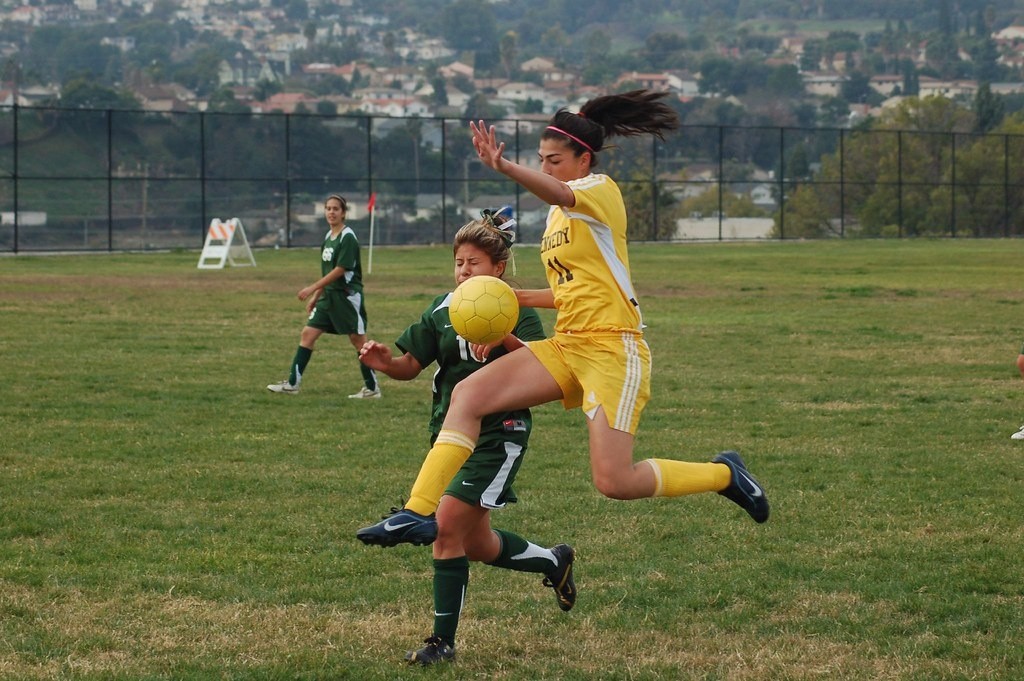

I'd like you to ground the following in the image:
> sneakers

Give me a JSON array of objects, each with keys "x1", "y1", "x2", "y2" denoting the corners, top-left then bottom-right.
[
  {"x1": 348, "y1": 387, "x2": 382, "y2": 399},
  {"x1": 267, "y1": 380, "x2": 299, "y2": 395},
  {"x1": 710, "y1": 449, "x2": 769, "y2": 523},
  {"x1": 403, "y1": 636, "x2": 456, "y2": 665},
  {"x1": 357, "y1": 494, "x2": 438, "y2": 548},
  {"x1": 542, "y1": 543, "x2": 576, "y2": 612}
]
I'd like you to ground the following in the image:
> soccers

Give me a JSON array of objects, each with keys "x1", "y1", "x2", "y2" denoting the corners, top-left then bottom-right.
[{"x1": 448, "y1": 275, "x2": 521, "y2": 346}]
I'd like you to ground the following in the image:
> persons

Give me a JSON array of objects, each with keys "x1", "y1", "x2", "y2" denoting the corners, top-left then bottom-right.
[
  {"x1": 359, "y1": 211, "x2": 577, "y2": 668},
  {"x1": 357, "y1": 88, "x2": 771, "y2": 548},
  {"x1": 266, "y1": 194, "x2": 382, "y2": 398}
]
